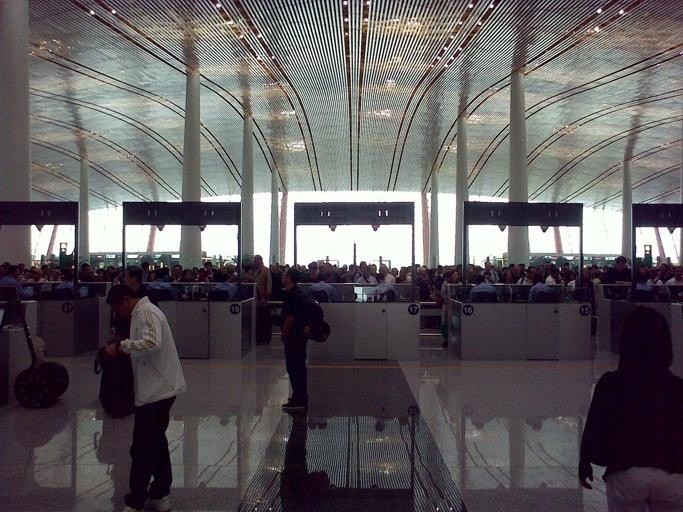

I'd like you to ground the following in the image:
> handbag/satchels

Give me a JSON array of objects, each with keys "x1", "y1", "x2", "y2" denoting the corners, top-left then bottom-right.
[{"x1": 99, "y1": 352, "x2": 134, "y2": 419}]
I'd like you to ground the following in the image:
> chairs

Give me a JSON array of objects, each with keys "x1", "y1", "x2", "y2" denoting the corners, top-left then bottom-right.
[
  {"x1": 535, "y1": 290, "x2": 560, "y2": 304},
  {"x1": 471, "y1": 291, "x2": 496, "y2": 303},
  {"x1": 379, "y1": 289, "x2": 396, "y2": 304},
  {"x1": 0, "y1": 283, "x2": 20, "y2": 303},
  {"x1": 146, "y1": 287, "x2": 177, "y2": 301},
  {"x1": 637, "y1": 289, "x2": 653, "y2": 301},
  {"x1": 207, "y1": 288, "x2": 230, "y2": 301},
  {"x1": 41, "y1": 287, "x2": 72, "y2": 301},
  {"x1": 310, "y1": 289, "x2": 328, "y2": 302}
]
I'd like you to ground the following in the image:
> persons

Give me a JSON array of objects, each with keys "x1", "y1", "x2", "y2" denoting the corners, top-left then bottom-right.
[
  {"x1": 0, "y1": 255, "x2": 124, "y2": 298},
  {"x1": 145, "y1": 254, "x2": 275, "y2": 346},
  {"x1": 105, "y1": 283, "x2": 187, "y2": 512},
  {"x1": 579, "y1": 304, "x2": 683, "y2": 512},
  {"x1": 114, "y1": 266, "x2": 159, "y2": 336},
  {"x1": 272, "y1": 254, "x2": 683, "y2": 303},
  {"x1": 279, "y1": 410, "x2": 307, "y2": 512},
  {"x1": 280, "y1": 272, "x2": 311, "y2": 409}
]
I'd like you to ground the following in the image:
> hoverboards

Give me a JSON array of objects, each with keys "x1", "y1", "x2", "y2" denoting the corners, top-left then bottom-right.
[
  {"x1": 7, "y1": 290, "x2": 69, "y2": 409},
  {"x1": 6, "y1": 401, "x2": 68, "y2": 508}
]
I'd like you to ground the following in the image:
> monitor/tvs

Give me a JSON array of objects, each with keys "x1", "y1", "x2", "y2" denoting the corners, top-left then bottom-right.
[
  {"x1": 77, "y1": 284, "x2": 91, "y2": 298},
  {"x1": 22, "y1": 284, "x2": 36, "y2": 299}
]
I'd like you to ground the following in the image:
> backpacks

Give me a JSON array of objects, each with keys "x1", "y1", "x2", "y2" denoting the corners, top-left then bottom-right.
[{"x1": 302, "y1": 298, "x2": 330, "y2": 343}]
